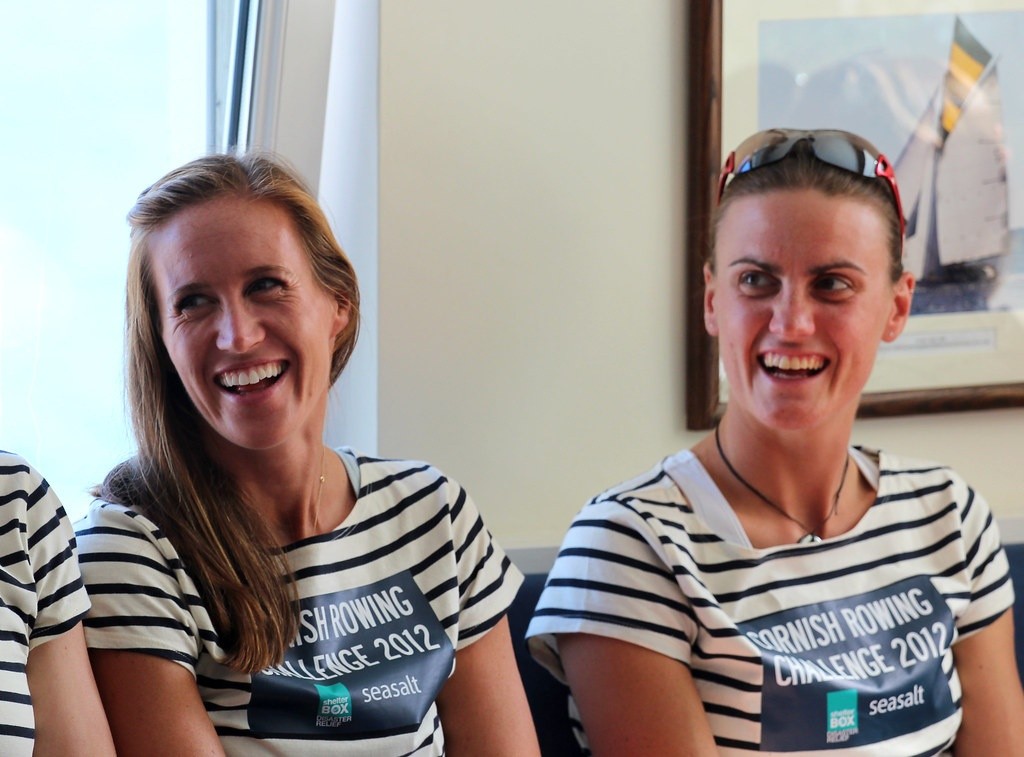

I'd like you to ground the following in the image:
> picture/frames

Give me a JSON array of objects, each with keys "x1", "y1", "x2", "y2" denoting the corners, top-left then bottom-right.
[{"x1": 685, "y1": 0, "x2": 1024, "y2": 431}]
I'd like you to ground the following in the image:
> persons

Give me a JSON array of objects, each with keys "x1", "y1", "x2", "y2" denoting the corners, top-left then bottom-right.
[
  {"x1": 71, "y1": 150, "x2": 541, "y2": 757},
  {"x1": 0, "y1": 449, "x2": 117, "y2": 757},
  {"x1": 521, "y1": 129, "x2": 1024, "y2": 757}
]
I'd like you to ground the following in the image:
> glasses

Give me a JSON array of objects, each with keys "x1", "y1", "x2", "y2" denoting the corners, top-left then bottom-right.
[{"x1": 716, "y1": 128, "x2": 905, "y2": 244}]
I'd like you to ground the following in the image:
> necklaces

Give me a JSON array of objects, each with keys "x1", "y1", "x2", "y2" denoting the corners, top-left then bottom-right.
[
  {"x1": 716, "y1": 419, "x2": 849, "y2": 547},
  {"x1": 253, "y1": 449, "x2": 327, "y2": 544}
]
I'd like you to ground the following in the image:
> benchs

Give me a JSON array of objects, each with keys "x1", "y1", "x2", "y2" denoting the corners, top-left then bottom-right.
[{"x1": 508, "y1": 544, "x2": 1024, "y2": 757}]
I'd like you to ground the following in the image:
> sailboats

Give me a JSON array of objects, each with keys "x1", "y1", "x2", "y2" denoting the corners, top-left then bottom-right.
[{"x1": 870, "y1": 11, "x2": 1011, "y2": 292}]
